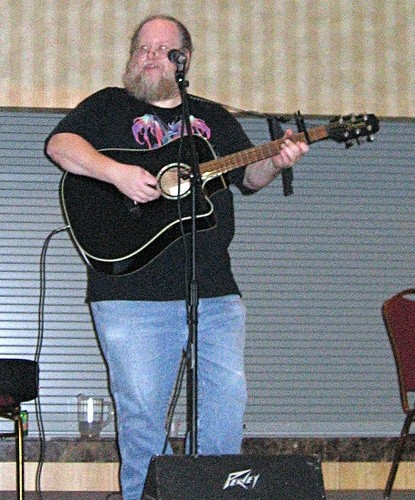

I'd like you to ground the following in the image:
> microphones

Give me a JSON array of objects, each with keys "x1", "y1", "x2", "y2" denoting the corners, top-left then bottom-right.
[{"x1": 167, "y1": 49, "x2": 187, "y2": 64}]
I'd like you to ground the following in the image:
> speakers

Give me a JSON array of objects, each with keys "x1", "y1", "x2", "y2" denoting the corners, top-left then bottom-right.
[{"x1": 139, "y1": 453, "x2": 326, "y2": 500}]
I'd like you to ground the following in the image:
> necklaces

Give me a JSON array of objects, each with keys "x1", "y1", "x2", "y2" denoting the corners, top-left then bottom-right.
[{"x1": 149, "y1": 104, "x2": 181, "y2": 144}]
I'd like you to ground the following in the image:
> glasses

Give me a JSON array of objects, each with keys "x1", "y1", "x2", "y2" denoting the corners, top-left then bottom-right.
[{"x1": 131, "y1": 47, "x2": 171, "y2": 59}]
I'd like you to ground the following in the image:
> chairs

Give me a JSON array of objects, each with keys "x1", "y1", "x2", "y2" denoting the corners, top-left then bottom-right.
[
  {"x1": 0, "y1": 358, "x2": 39, "y2": 500},
  {"x1": 381, "y1": 289, "x2": 415, "y2": 500}
]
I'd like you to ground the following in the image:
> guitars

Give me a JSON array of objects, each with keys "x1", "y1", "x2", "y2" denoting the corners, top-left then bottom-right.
[{"x1": 60, "y1": 112, "x2": 379, "y2": 278}]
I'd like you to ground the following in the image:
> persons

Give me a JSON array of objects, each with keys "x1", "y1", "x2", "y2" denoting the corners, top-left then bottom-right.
[{"x1": 43, "y1": 11, "x2": 311, "y2": 500}]
